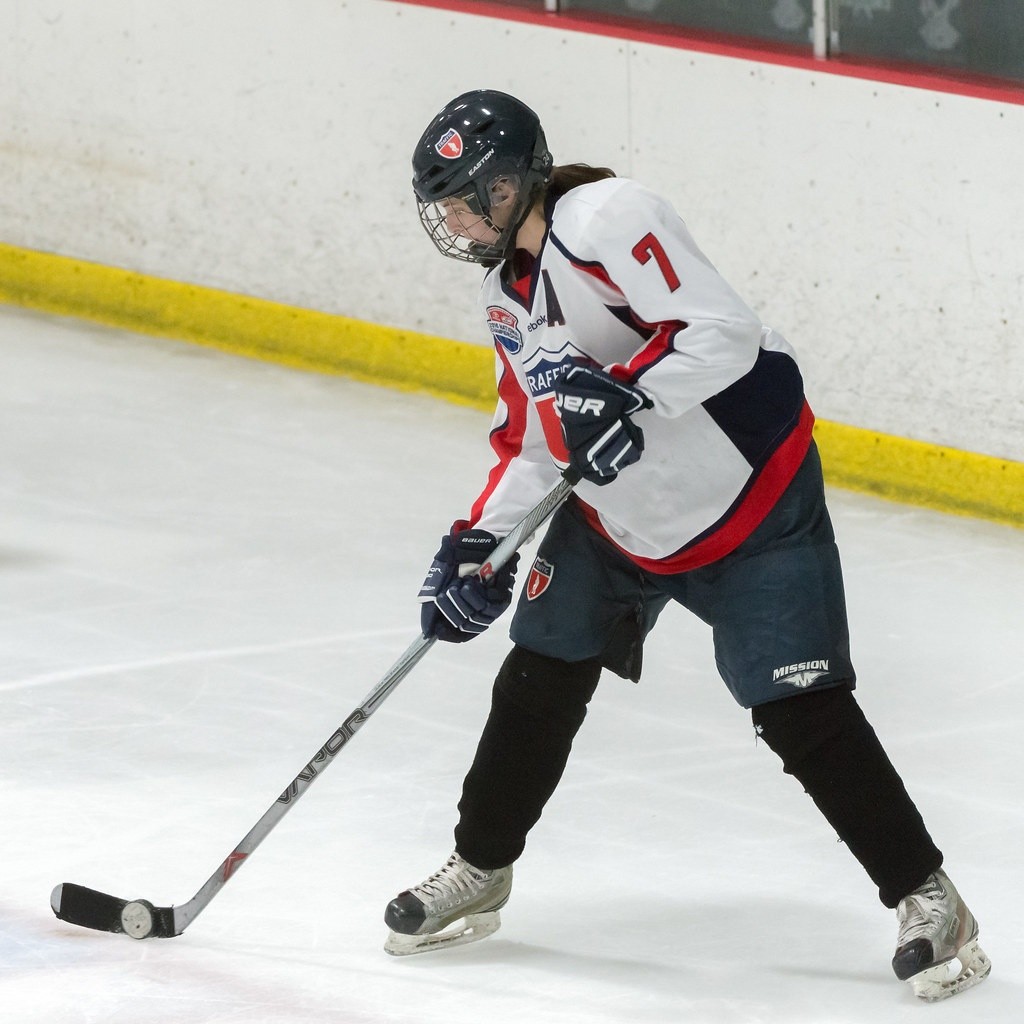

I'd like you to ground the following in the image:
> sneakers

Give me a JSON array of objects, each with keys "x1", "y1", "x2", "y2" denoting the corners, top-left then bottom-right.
[
  {"x1": 384, "y1": 854, "x2": 515, "y2": 955},
  {"x1": 892, "y1": 869, "x2": 993, "y2": 1001}
]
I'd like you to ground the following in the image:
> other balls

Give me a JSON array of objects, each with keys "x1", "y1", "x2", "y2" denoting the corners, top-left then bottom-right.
[{"x1": 119, "y1": 899, "x2": 160, "y2": 940}]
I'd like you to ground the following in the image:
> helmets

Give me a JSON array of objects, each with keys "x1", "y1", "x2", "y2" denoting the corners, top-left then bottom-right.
[{"x1": 412, "y1": 91, "x2": 551, "y2": 266}]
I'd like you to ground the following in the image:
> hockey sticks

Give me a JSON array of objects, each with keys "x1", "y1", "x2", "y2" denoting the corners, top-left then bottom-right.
[{"x1": 48, "y1": 458, "x2": 598, "y2": 939}]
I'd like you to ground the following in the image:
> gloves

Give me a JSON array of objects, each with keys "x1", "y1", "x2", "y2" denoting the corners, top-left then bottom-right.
[
  {"x1": 419, "y1": 530, "x2": 519, "y2": 645},
  {"x1": 555, "y1": 356, "x2": 654, "y2": 484}
]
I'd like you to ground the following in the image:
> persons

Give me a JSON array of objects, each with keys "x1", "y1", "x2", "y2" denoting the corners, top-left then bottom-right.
[{"x1": 381, "y1": 90, "x2": 993, "y2": 1002}]
